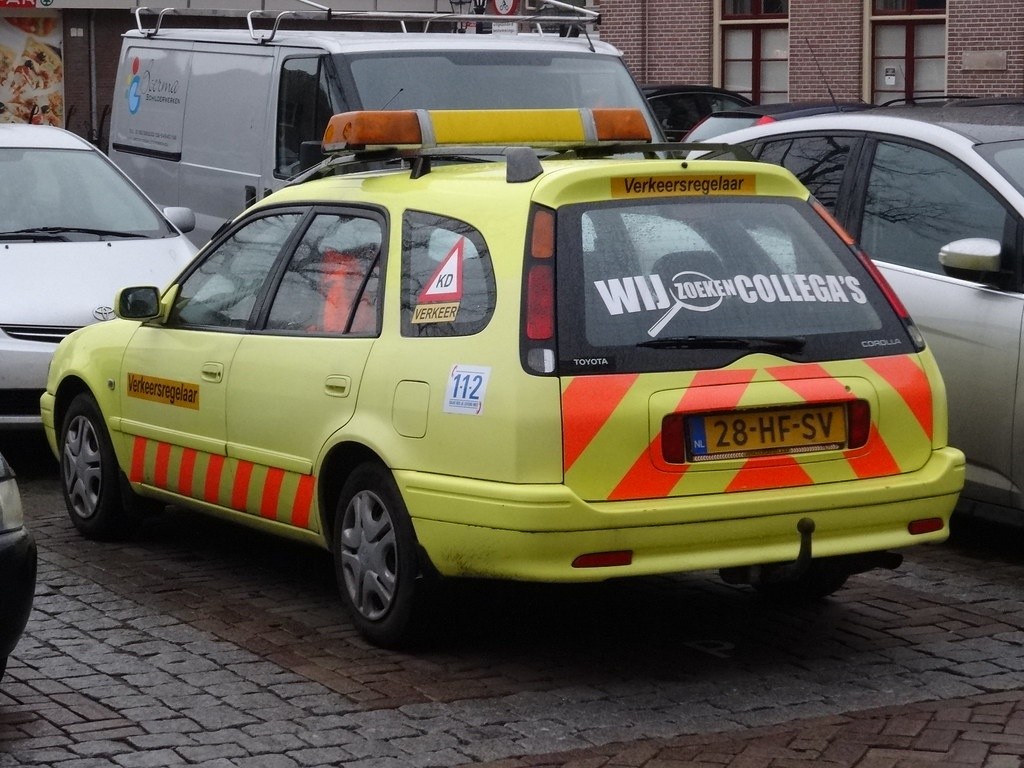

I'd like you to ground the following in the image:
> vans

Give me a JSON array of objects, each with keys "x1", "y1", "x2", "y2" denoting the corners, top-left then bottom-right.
[{"x1": 99, "y1": 0, "x2": 670, "y2": 251}]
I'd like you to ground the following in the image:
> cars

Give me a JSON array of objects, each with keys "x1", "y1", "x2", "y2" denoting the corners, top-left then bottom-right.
[
  {"x1": 680, "y1": 93, "x2": 1024, "y2": 534},
  {"x1": 39, "y1": 103, "x2": 969, "y2": 650},
  {"x1": 0, "y1": 119, "x2": 210, "y2": 434},
  {"x1": 634, "y1": 83, "x2": 759, "y2": 151}
]
